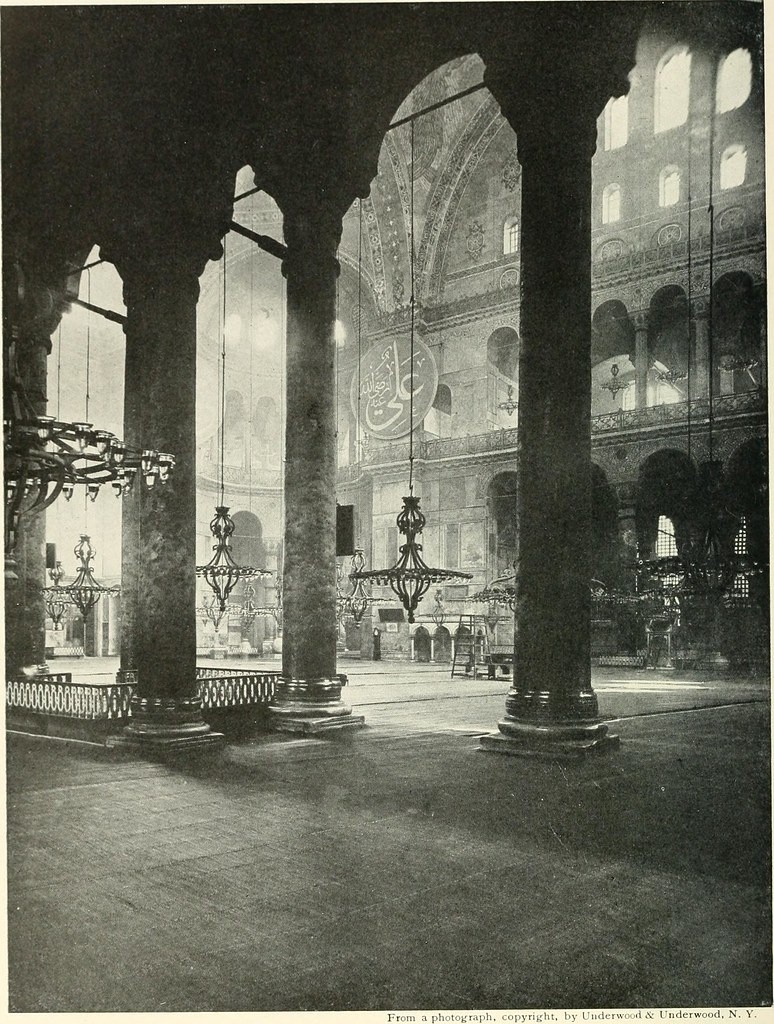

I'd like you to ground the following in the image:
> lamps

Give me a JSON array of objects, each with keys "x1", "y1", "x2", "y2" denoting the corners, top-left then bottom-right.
[
  {"x1": 587, "y1": 36, "x2": 770, "y2": 651},
  {"x1": 45, "y1": 267, "x2": 120, "y2": 631},
  {"x1": 336, "y1": 119, "x2": 515, "y2": 633},
  {"x1": 1, "y1": 322, "x2": 178, "y2": 581},
  {"x1": 195, "y1": 235, "x2": 285, "y2": 634}
]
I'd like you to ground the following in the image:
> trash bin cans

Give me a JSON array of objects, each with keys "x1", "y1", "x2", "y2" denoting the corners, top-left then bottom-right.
[{"x1": 488, "y1": 664, "x2": 503, "y2": 680}]
